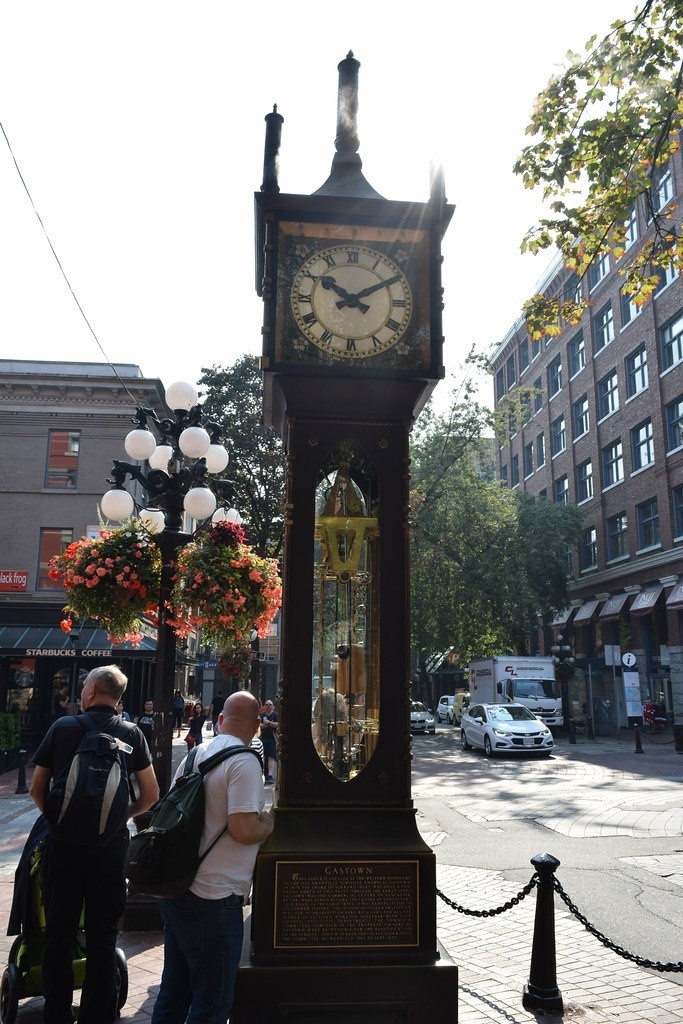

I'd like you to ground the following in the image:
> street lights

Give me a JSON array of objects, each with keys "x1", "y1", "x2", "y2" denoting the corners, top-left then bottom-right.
[
  {"x1": 548, "y1": 636, "x2": 580, "y2": 744},
  {"x1": 98, "y1": 381, "x2": 244, "y2": 930}
]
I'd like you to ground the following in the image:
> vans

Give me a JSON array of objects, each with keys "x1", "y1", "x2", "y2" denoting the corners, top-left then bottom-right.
[{"x1": 453, "y1": 693, "x2": 469, "y2": 724}]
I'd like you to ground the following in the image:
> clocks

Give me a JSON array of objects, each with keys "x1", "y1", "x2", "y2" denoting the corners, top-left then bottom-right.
[{"x1": 289, "y1": 243, "x2": 414, "y2": 359}]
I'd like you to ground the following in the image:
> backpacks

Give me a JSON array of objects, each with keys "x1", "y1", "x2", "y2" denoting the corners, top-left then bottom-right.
[
  {"x1": 52, "y1": 712, "x2": 138, "y2": 851},
  {"x1": 124, "y1": 745, "x2": 264, "y2": 897}
]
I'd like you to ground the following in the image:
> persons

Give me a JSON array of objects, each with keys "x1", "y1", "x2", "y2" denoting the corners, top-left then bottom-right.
[
  {"x1": 648, "y1": 699, "x2": 669, "y2": 721},
  {"x1": 29, "y1": 664, "x2": 162, "y2": 1024},
  {"x1": 123, "y1": 688, "x2": 280, "y2": 1024}
]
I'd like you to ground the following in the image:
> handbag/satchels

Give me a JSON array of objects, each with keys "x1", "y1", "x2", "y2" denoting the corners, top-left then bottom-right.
[{"x1": 184, "y1": 734, "x2": 197, "y2": 744}]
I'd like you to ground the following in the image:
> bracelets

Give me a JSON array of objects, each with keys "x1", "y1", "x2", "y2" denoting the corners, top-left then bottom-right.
[{"x1": 266, "y1": 720, "x2": 271, "y2": 725}]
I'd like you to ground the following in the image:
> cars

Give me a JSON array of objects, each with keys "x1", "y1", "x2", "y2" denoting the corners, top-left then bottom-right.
[
  {"x1": 571, "y1": 701, "x2": 587, "y2": 734},
  {"x1": 459, "y1": 703, "x2": 554, "y2": 759},
  {"x1": 435, "y1": 695, "x2": 455, "y2": 724},
  {"x1": 410, "y1": 701, "x2": 435, "y2": 735}
]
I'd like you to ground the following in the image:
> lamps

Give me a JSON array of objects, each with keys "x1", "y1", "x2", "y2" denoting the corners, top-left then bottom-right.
[{"x1": 70, "y1": 631, "x2": 81, "y2": 641}]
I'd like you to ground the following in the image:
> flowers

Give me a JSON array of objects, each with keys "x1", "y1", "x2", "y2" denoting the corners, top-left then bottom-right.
[{"x1": 47, "y1": 503, "x2": 283, "y2": 683}]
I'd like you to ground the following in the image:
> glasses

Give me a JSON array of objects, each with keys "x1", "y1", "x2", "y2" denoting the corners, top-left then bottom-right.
[{"x1": 265, "y1": 704, "x2": 273, "y2": 706}]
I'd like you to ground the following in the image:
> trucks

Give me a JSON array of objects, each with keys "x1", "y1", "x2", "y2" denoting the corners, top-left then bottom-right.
[{"x1": 468, "y1": 658, "x2": 565, "y2": 740}]
